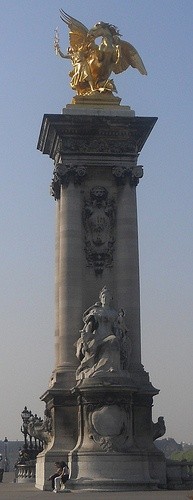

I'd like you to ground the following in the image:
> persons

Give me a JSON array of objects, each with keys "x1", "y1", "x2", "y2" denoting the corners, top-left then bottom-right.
[
  {"x1": 54, "y1": 44, "x2": 91, "y2": 83},
  {"x1": 76, "y1": 285, "x2": 130, "y2": 373},
  {"x1": 51, "y1": 461, "x2": 69, "y2": 493},
  {"x1": 47, "y1": 461, "x2": 63, "y2": 492},
  {"x1": 0, "y1": 453, "x2": 8, "y2": 483}
]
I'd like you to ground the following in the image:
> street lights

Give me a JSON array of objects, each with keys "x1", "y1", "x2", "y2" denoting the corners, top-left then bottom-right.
[
  {"x1": 2, "y1": 437, "x2": 9, "y2": 472},
  {"x1": 19, "y1": 406, "x2": 43, "y2": 451}
]
